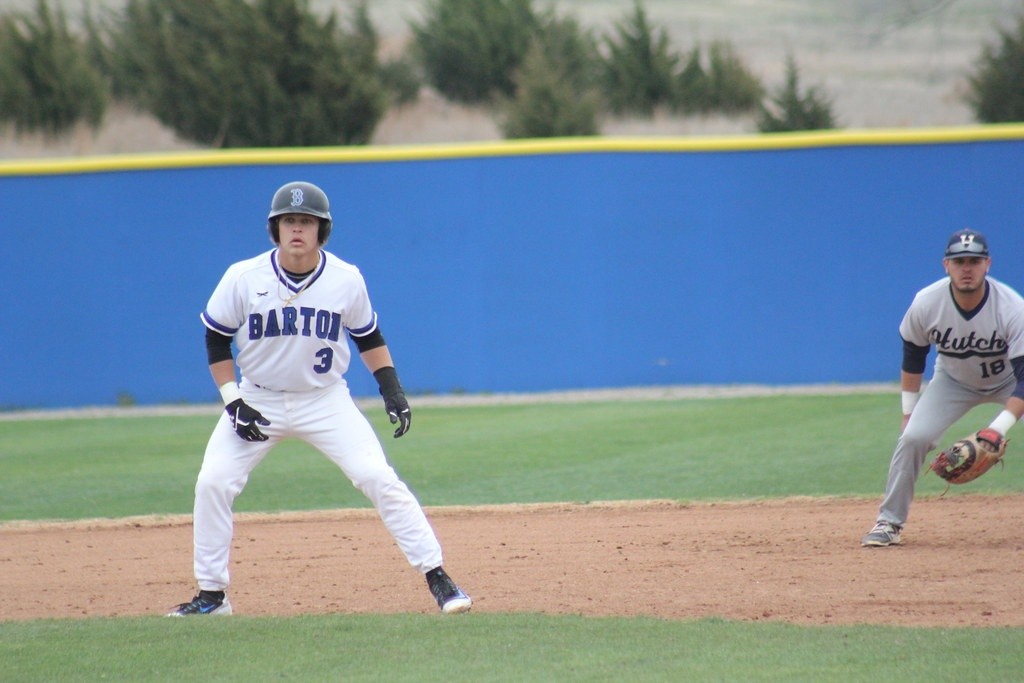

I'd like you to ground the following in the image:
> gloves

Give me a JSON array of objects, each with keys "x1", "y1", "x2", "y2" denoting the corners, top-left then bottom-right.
[
  {"x1": 225, "y1": 398, "x2": 271, "y2": 441},
  {"x1": 385, "y1": 392, "x2": 411, "y2": 439}
]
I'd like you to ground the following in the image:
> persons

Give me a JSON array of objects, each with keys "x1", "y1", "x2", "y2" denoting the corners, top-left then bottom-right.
[
  {"x1": 164, "y1": 181, "x2": 473, "y2": 617},
  {"x1": 860, "y1": 230, "x2": 1024, "y2": 547}
]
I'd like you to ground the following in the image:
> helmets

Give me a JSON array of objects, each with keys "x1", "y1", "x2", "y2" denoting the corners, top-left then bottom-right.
[{"x1": 267, "y1": 181, "x2": 332, "y2": 243}]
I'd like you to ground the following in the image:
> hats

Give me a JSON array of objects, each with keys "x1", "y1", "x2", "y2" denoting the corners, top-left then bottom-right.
[{"x1": 944, "y1": 229, "x2": 989, "y2": 260}]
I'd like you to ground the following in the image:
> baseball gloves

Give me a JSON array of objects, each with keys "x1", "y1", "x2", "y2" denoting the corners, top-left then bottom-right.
[{"x1": 929, "y1": 429, "x2": 1008, "y2": 485}]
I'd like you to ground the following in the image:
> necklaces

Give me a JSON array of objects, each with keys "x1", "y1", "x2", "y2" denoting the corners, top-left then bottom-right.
[{"x1": 277, "y1": 254, "x2": 320, "y2": 308}]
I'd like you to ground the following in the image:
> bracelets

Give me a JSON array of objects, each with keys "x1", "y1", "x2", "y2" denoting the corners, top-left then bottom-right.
[
  {"x1": 219, "y1": 381, "x2": 240, "y2": 406},
  {"x1": 901, "y1": 391, "x2": 919, "y2": 414},
  {"x1": 989, "y1": 410, "x2": 1016, "y2": 436}
]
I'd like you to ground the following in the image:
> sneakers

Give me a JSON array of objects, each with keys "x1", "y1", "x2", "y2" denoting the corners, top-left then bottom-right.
[
  {"x1": 426, "y1": 565, "x2": 471, "y2": 614},
  {"x1": 165, "y1": 591, "x2": 233, "y2": 617},
  {"x1": 860, "y1": 520, "x2": 904, "y2": 548}
]
